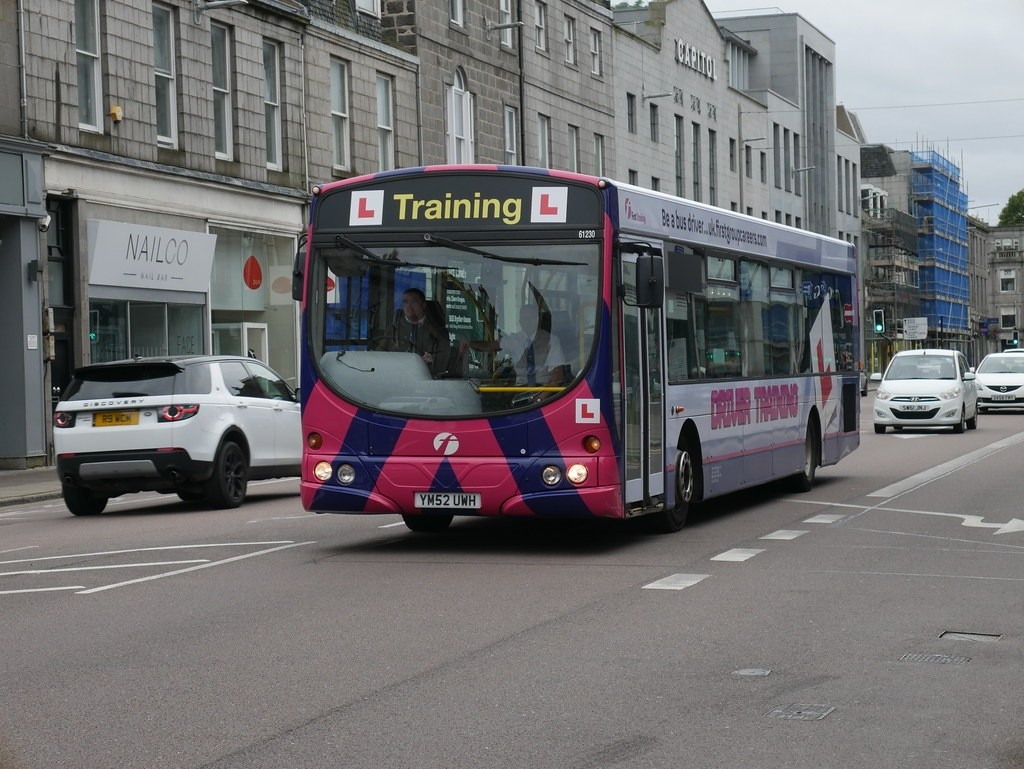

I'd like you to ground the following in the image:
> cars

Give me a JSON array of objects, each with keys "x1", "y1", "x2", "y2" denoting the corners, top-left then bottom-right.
[
  {"x1": 970, "y1": 352, "x2": 1024, "y2": 414},
  {"x1": 870, "y1": 349, "x2": 979, "y2": 434}
]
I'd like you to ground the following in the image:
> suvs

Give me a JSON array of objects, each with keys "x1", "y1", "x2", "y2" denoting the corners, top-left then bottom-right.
[{"x1": 53, "y1": 353, "x2": 304, "y2": 517}]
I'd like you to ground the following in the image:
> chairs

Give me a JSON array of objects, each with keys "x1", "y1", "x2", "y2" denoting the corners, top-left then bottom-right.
[{"x1": 541, "y1": 311, "x2": 577, "y2": 364}]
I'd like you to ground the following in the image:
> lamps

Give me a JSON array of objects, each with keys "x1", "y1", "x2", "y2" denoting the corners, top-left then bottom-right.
[
  {"x1": 741, "y1": 136, "x2": 767, "y2": 143},
  {"x1": 790, "y1": 164, "x2": 815, "y2": 178},
  {"x1": 872, "y1": 309, "x2": 886, "y2": 334},
  {"x1": 640, "y1": 83, "x2": 673, "y2": 107},
  {"x1": 1013, "y1": 331, "x2": 1020, "y2": 345},
  {"x1": 484, "y1": 14, "x2": 525, "y2": 41}
]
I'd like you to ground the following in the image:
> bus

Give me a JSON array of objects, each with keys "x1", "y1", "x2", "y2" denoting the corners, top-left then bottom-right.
[{"x1": 290, "y1": 162, "x2": 862, "y2": 547}]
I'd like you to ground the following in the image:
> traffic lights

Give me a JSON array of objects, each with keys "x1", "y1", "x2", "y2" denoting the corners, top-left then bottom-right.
[
  {"x1": 1012, "y1": 330, "x2": 1019, "y2": 344},
  {"x1": 873, "y1": 310, "x2": 885, "y2": 334}
]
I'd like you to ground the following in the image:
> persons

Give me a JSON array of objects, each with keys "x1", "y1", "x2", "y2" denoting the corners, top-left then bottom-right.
[
  {"x1": 374, "y1": 286, "x2": 452, "y2": 375},
  {"x1": 464, "y1": 304, "x2": 568, "y2": 408}
]
